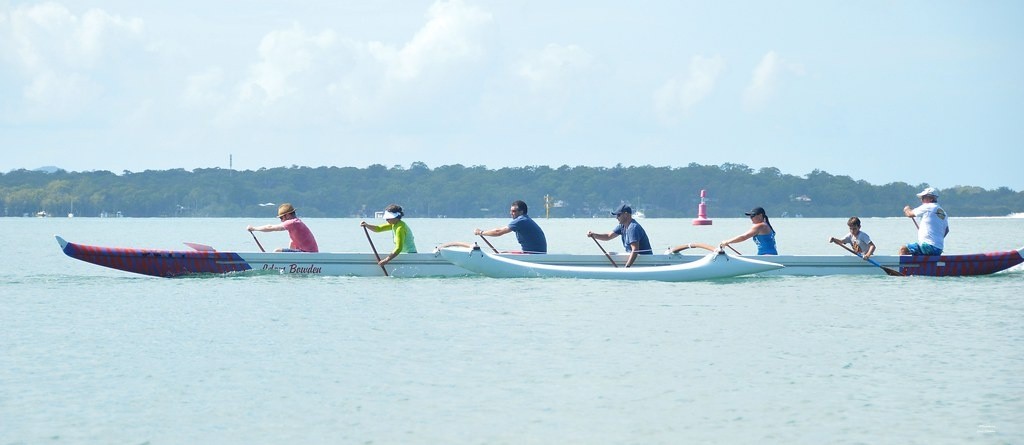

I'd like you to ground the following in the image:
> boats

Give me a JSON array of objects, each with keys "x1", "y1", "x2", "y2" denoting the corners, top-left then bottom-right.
[
  {"x1": 54, "y1": 233, "x2": 1024, "y2": 286},
  {"x1": 432, "y1": 241, "x2": 787, "y2": 281}
]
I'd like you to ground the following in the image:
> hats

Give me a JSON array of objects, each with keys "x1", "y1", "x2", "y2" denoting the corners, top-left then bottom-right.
[
  {"x1": 611, "y1": 204, "x2": 632, "y2": 215},
  {"x1": 745, "y1": 207, "x2": 765, "y2": 216},
  {"x1": 383, "y1": 210, "x2": 401, "y2": 219},
  {"x1": 277, "y1": 203, "x2": 296, "y2": 217},
  {"x1": 916, "y1": 187, "x2": 939, "y2": 199}
]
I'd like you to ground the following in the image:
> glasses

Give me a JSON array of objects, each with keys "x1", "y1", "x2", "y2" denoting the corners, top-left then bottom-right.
[
  {"x1": 280, "y1": 217, "x2": 284, "y2": 219},
  {"x1": 511, "y1": 210, "x2": 520, "y2": 213},
  {"x1": 617, "y1": 214, "x2": 623, "y2": 217},
  {"x1": 850, "y1": 226, "x2": 860, "y2": 231},
  {"x1": 751, "y1": 215, "x2": 757, "y2": 219},
  {"x1": 921, "y1": 196, "x2": 930, "y2": 199}
]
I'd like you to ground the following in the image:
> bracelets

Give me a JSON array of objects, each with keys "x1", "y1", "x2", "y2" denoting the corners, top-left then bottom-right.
[
  {"x1": 626, "y1": 266, "x2": 630, "y2": 267},
  {"x1": 481, "y1": 231, "x2": 483, "y2": 234},
  {"x1": 866, "y1": 255, "x2": 868, "y2": 258}
]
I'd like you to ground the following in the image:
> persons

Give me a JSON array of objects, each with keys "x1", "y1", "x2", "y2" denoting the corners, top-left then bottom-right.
[
  {"x1": 473, "y1": 200, "x2": 547, "y2": 254},
  {"x1": 586, "y1": 204, "x2": 653, "y2": 268},
  {"x1": 899, "y1": 187, "x2": 949, "y2": 256},
  {"x1": 360, "y1": 204, "x2": 417, "y2": 268},
  {"x1": 720, "y1": 206, "x2": 778, "y2": 255},
  {"x1": 830, "y1": 218, "x2": 876, "y2": 260},
  {"x1": 247, "y1": 203, "x2": 318, "y2": 252}
]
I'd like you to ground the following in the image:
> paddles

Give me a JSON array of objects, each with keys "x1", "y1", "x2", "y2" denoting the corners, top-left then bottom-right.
[
  {"x1": 590, "y1": 235, "x2": 618, "y2": 267},
  {"x1": 912, "y1": 216, "x2": 919, "y2": 229},
  {"x1": 481, "y1": 235, "x2": 498, "y2": 253},
  {"x1": 727, "y1": 244, "x2": 742, "y2": 256},
  {"x1": 249, "y1": 229, "x2": 266, "y2": 252},
  {"x1": 833, "y1": 239, "x2": 904, "y2": 276},
  {"x1": 363, "y1": 225, "x2": 388, "y2": 276}
]
